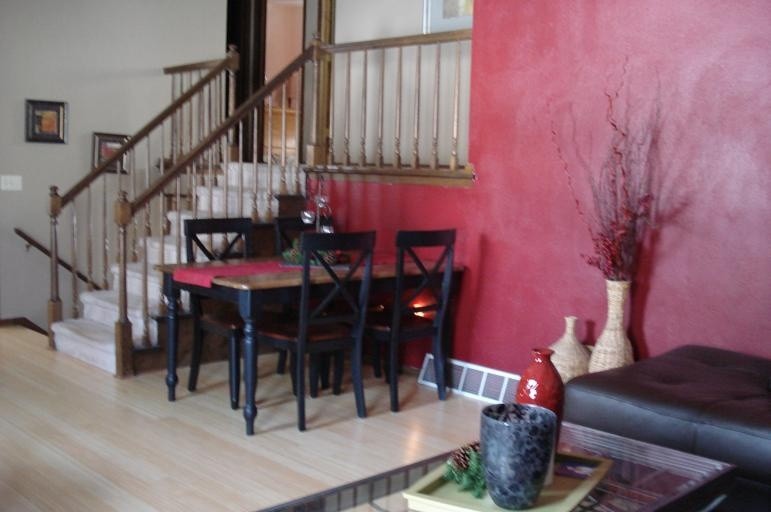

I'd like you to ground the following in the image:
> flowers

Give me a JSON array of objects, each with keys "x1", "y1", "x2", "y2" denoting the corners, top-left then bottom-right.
[{"x1": 552, "y1": 56, "x2": 670, "y2": 279}]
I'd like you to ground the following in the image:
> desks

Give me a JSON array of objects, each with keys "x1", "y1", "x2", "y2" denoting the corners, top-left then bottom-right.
[
  {"x1": 255, "y1": 418, "x2": 740, "y2": 512},
  {"x1": 153, "y1": 252, "x2": 470, "y2": 412}
]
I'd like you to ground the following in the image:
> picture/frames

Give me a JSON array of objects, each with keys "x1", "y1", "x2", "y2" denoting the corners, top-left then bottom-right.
[
  {"x1": 25, "y1": 98, "x2": 71, "y2": 144},
  {"x1": 92, "y1": 132, "x2": 133, "y2": 175},
  {"x1": 422, "y1": 0, "x2": 475, "y2": 37}
]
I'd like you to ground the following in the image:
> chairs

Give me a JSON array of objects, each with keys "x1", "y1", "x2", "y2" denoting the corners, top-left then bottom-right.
[
  {"x1": 180, "y1": 214, "x2": 301, "y2": 410},
  {"x1": 252, "y1": 228, "x2": 379, "y2": 429},
  {"x1": 272, "y1": 213, "x2": 376, "y2": 376},
  {"x1": 332, "y1": 222, "x2": 461, "y2": 410}
]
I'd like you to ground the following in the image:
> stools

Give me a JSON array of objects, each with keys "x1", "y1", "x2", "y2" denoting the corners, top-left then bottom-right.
[{"x1": 563, "y1": 343, "x2": 771, "y2": 510}]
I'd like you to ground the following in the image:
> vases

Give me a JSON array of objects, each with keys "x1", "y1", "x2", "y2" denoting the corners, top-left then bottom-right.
[
  {"x1": 548, "y1": 312, "x2": 593, "y2": 385},
  {"x1": 588, "y1": 277, "x2": 637, "y2": 374},
  {"x1": 517, "y1": 346, "x2": 567, "y2": 420}
]
